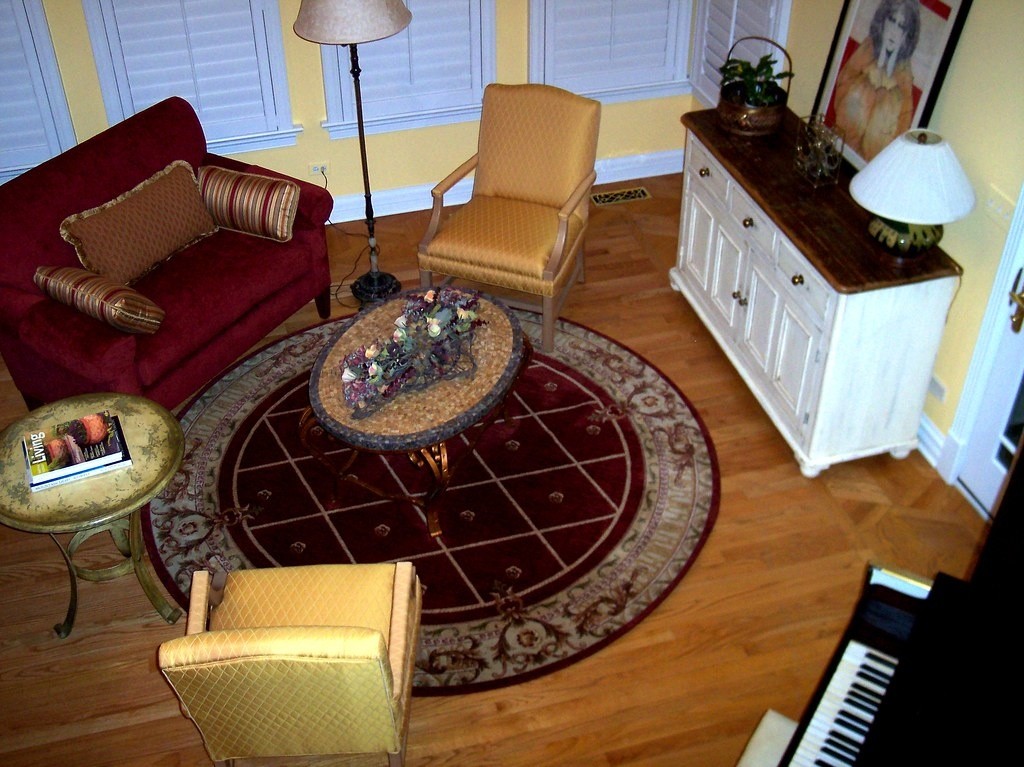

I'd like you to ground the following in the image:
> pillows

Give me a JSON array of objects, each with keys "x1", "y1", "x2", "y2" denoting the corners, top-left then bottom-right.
[
  {"x1": 33, "y1": 265, "x2": 167, "y2": 336},
  {"x1": 197, "y1": 164, "x2": 300, "y2": 243},
  {"x1": 59, "y1": 160, "x2": 219, "y2": 285}
]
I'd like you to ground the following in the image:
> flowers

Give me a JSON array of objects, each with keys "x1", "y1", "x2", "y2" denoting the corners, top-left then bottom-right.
[{"x1": 338, "y1": 284, "x2": 490, "y2": 420}]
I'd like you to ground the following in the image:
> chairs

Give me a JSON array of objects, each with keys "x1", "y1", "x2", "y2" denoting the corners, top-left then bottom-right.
[
  {"x1": 417, "y1": 82, "x2": 602, "y2": 352},
  {"x1": 157, "y1": 561, "x2": 423, "y2": 767}
]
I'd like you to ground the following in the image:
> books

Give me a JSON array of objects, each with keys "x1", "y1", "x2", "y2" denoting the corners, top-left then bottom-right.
[{"x1": 22, "y1": 409, "x2": 133, "y2": 493}]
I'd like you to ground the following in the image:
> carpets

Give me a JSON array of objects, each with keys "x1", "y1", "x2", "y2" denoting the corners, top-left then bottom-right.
[{"x1": 126, "y1": 299, "x2": 721, "y2": 697}]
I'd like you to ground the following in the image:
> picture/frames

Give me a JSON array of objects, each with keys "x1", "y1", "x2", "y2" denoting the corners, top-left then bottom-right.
[{"x1": 808, "y1": 0, "x2": 974, "y2": 180}]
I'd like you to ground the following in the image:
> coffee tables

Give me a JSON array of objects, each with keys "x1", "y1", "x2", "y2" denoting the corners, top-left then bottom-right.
[{"x1": 310, "y1": 286, "x2": 524, "y2": 538}]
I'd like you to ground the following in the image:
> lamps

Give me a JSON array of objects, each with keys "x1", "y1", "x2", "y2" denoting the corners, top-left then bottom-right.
[
  {"x1": 292, "y1": 0, "x2": 412, "y2": 305},
  {"x1": 849, "y1": 128, "x2": 977, "y2": 256}
]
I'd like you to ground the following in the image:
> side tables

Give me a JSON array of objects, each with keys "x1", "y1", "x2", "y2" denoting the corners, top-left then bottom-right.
[{"x1": 0, "y1": 393, "x2": 183, "y2": 639}]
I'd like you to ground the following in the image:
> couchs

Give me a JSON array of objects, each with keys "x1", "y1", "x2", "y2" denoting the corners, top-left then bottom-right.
[{"x1": 0, "y1": 94, "x2": 332, "y2": 412}]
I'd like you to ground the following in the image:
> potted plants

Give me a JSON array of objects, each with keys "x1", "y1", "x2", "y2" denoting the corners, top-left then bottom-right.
[{"x1": 716, "y1": 35, "x2": 795, "y2": 138}]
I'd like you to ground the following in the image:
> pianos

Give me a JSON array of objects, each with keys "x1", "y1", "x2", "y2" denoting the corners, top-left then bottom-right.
[{"x1": 775, "y1": 421, "x2": 1024, "y2": 767}]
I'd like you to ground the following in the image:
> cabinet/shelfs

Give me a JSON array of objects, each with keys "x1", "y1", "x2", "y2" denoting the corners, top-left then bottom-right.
[{"x1": 669, "y1": 105, "x2": 963, "y2": 477}]
